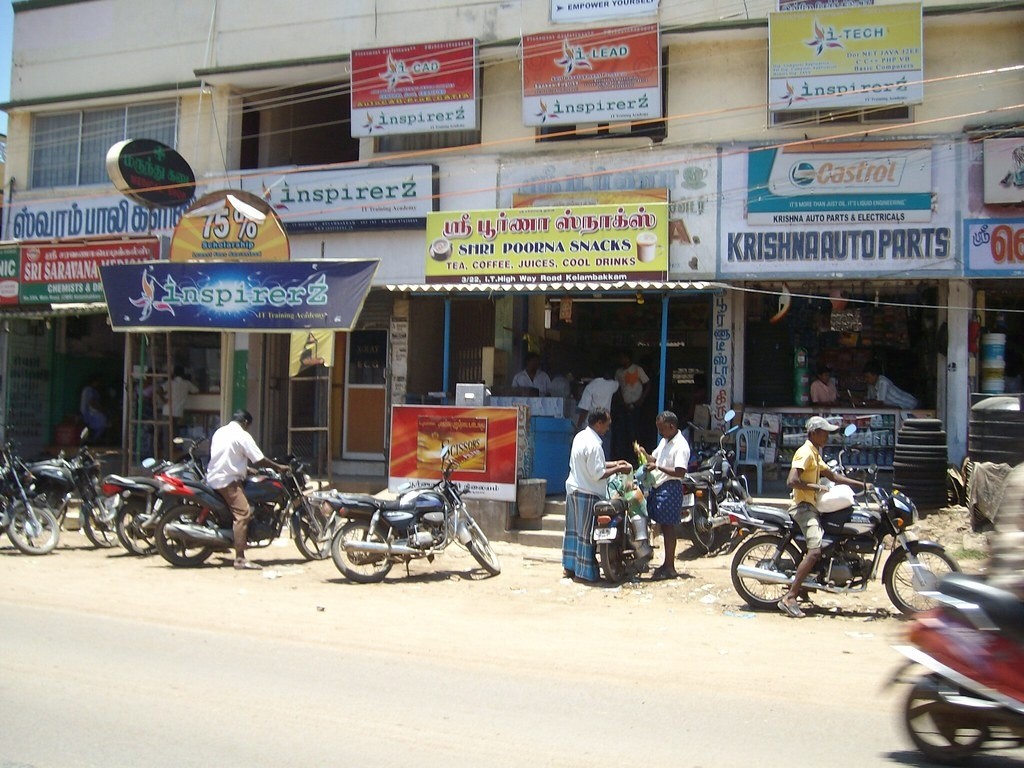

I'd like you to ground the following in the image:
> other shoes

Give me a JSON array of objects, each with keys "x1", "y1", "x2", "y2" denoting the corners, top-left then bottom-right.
[{"x1": 563, "y1": 570, "x2": 592, "y2": 583}]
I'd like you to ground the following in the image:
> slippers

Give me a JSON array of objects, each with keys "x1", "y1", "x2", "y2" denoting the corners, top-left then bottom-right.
[
  {"x1": 778, "y1": 595, "x2": 805, "y2": 617},
  {"x1": 234, "y1": 559, "x2": 262, "y2": 569},
  {"x1": 650, "y1": 564, "x2": 679, "y2": 581}
]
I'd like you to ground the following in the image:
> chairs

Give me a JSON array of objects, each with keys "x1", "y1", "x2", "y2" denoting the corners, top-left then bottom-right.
[{"x1": 732, "y1": 426, "x2": 769, "y2": 497}]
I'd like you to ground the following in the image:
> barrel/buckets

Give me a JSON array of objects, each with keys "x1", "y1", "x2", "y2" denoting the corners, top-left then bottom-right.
[
  {"x1": 793, "y1": 345, "x2": 809, "y2": 408},
  {"x1": 982, "y1": 333, "x2": 1006, "y2": 394}
]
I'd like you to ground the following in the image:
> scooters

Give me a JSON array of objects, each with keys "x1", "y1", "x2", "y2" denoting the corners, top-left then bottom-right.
[{"x1": 881, "y1": 567, "x2": 1024, "y2": 762}]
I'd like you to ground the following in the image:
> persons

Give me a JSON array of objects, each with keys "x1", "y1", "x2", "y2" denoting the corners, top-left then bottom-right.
[
  {"x1": 992, "y1": 461, "x2": 1024, "y2": 600},
  {"x1": 136, "y1": 367, "x2": 199, "y2": 458},
  {"x1": 778, "y1": 415, "x2": 874, "y2": 617},
  {"x1": 82, "y1": 373, "x2": 111, "y2": 442},
  {"x1": 513, "y1": 350, "x2": 707, "y2": 460},
  {"x1": 635, "y1": 410, "x2": 690, "y2": 579},
  {"x1": 863, "y1": 360, "x2": 920, "y2": 411},
  {"x1": 811, "y1": 366, "x2": 841, "y2": 407},
  {"x1": 560, "y1": 407, "x2": 632, "y2": 583},
  {"x1": 206, "y1": 408, "x2": 292, "y2": 570}
]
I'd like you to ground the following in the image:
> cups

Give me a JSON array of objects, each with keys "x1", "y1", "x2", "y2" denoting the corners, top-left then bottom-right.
[
  {"x1": 637, "y1": 232, "x2": 657, "y2": 262},
  {"x1": 432, "y1": 239, "x2": 450, "y2": 255}
]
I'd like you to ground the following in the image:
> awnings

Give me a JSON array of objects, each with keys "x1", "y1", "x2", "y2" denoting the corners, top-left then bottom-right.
[{"x1": 384, "y1": 282, "x2": 724, "y2": 293}]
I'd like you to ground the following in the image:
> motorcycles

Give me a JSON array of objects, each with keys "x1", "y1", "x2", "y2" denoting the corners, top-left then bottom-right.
[
  {"x1": 312, "y1": 446, "x2": 500, "y2": 586},
  {"x1": 679, "y1": 410, "x2": 757, "y2": 552},
  {"x1": 2, "y1": 426, "x2": 335, "y2": 568},
  {"x1": 718, "y1": 461, "x2": 959, "y2": 616},
  {"x1": 821, "y1": 423, "x2": 866, "y2": 491},
  {"x1": 592, "y1": 471, "x2": 660, "y2": 583}
]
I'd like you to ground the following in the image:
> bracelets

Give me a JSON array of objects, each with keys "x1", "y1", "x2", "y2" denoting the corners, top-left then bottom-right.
[{"x1": 615, "y1": 461, "x2": 617, "y2": 467}]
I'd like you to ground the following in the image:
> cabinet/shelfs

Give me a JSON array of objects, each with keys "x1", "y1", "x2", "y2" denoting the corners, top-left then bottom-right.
[{"x1": 779, "y1": 409, "x2": 898, "y2": 474}]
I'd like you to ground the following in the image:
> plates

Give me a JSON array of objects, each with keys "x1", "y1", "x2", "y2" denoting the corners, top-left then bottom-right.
[{"x1": 429, "y1": 244, "x2": 452, "y2": 260}]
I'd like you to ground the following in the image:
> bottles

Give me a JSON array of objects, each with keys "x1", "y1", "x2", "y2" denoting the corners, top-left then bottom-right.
[{"x1": 781, "y1": 412, "x2": 895, "y2": 465}]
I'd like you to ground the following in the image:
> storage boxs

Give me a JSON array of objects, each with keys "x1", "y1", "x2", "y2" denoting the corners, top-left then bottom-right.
[
  {"x1": 754, "y1": 464, "x2": 779, "y2": 481},
  {"x1": 55, "y1": 424, "x2": 81, "y2": 446}
]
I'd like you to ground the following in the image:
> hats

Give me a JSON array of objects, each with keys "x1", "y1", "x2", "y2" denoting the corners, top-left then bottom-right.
[{"x1": 804, "y1": 416, "x2": 839, "y2": 433}]
